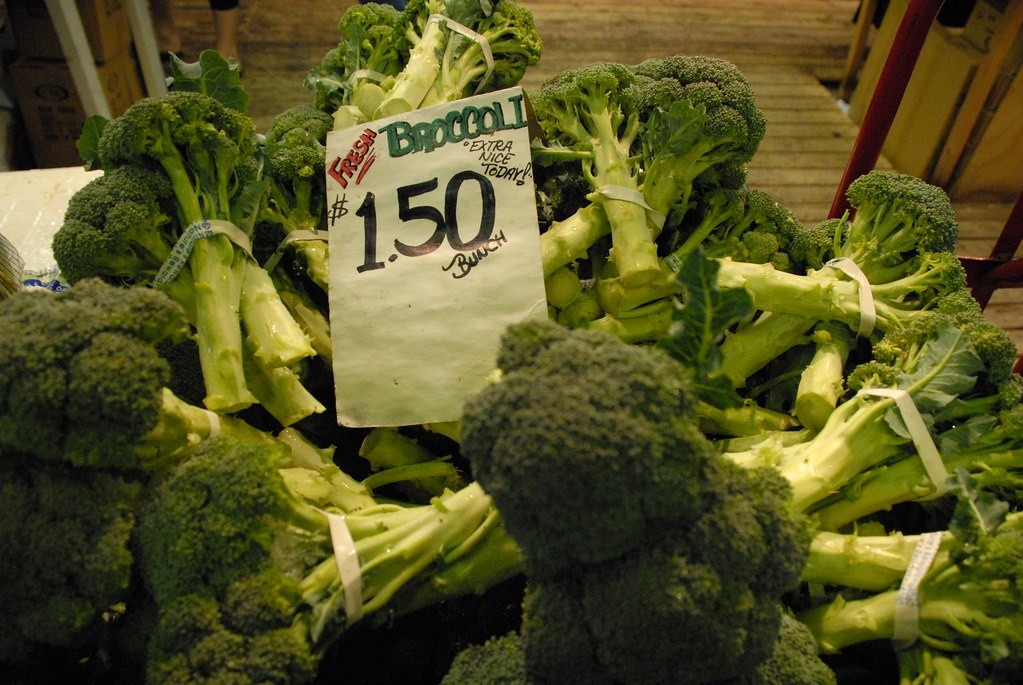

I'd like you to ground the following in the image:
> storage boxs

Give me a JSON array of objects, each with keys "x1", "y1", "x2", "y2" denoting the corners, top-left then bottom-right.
[
  {"x1": 8, "y1": 0, "x2": 131, "y2": 65},
  {"x1": 11, "y1": 53, "x2": 144, "y2": 169}
]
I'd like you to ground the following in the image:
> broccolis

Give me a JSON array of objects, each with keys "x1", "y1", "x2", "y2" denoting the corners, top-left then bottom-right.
[{"x1": 0, "y1": 0, "x2": 1023, "y2": 685}]
